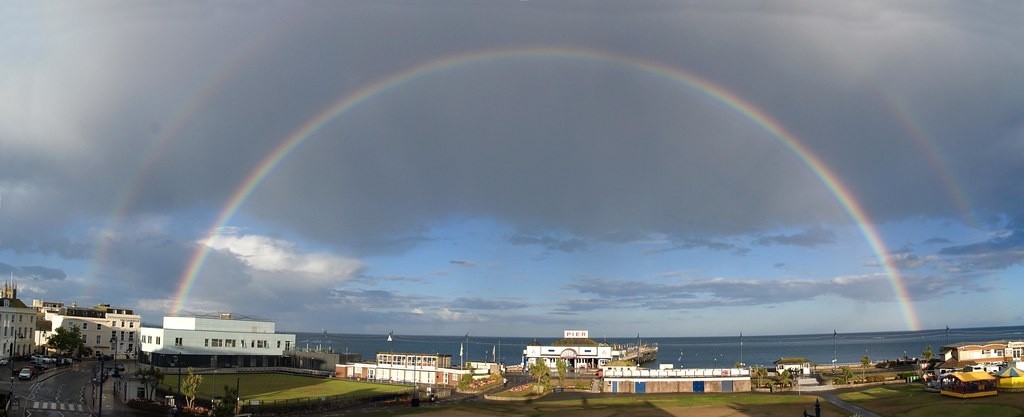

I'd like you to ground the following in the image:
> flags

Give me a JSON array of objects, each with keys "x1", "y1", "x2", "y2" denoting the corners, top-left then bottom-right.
[
  {"x1": 492, "y1": 345, "x2": 495, "y2": 355},
  {"x1": 460, "y1": 343, "x2": 463, "y2": 356}
]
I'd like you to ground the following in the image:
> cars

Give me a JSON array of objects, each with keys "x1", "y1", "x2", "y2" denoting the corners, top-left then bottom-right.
[
  {"x1": 93, "y1": 367, "x2": 108, "y2": 383},
  {"x1": 14, "y1": 355, "x2": 73, "y2": 381}
]
